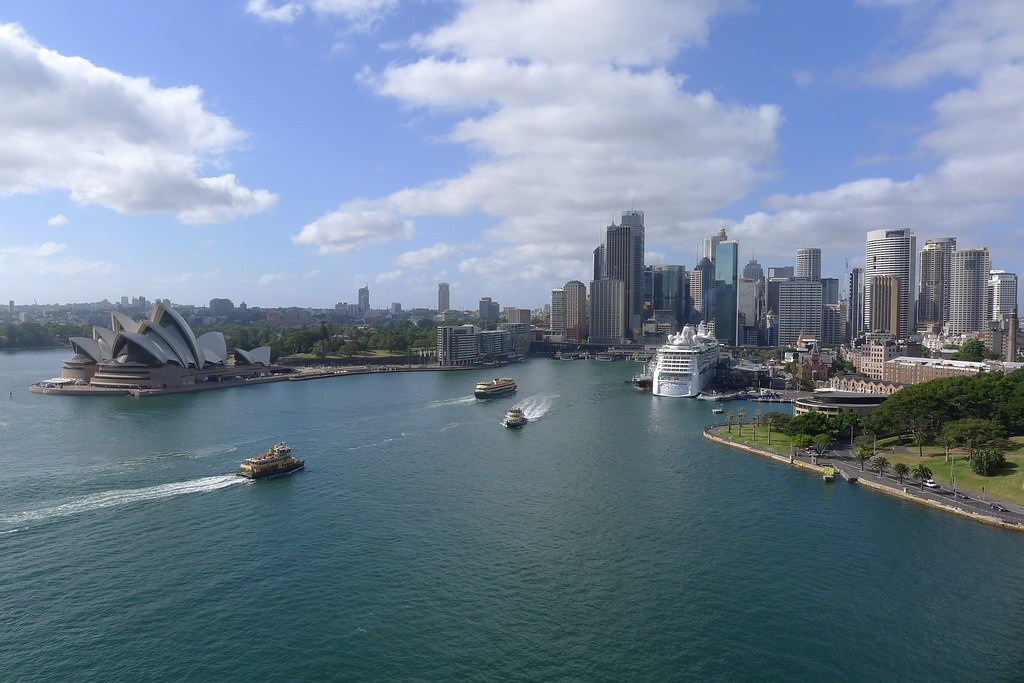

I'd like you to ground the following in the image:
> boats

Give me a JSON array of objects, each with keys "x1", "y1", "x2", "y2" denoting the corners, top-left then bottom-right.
[
  {"x1": 236, "y1": 441, "x2": 305, "y2": 480},
  {"x1": 474, "y1": 374, "x2": 517, "y2": 401},
  {"x1": 505, "y1": 405, "x2": 527, "y2": 429}
]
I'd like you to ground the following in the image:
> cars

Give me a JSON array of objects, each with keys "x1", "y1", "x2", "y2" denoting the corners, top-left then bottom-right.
[{"x1": 920, "y1": 479, "x2": 937, "y2": 488}]
